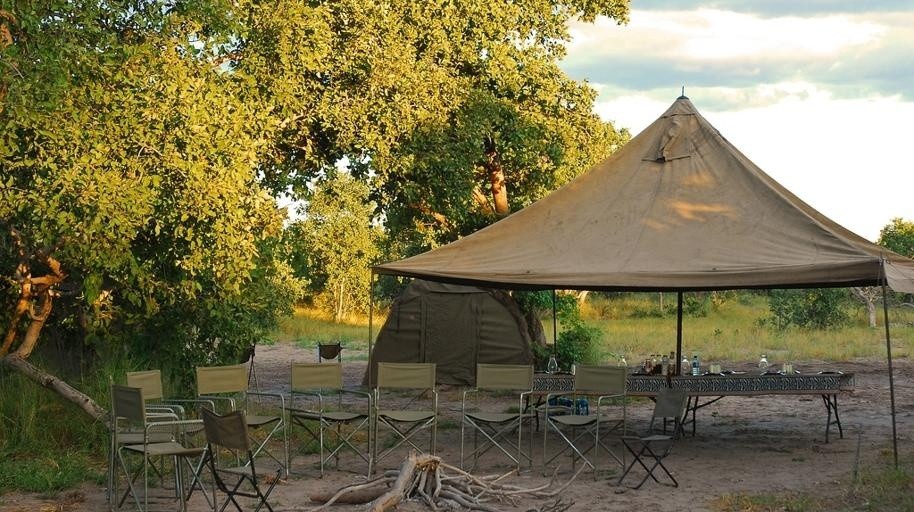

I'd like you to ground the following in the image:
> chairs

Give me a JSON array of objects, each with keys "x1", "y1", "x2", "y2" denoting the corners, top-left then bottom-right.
[{"x1": 318, "y1": 342, "x2": 344, "y2": 362}]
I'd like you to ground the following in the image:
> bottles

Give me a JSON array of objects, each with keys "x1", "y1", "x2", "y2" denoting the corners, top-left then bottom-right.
[
  {"x1": 782, "y1": 361, "x2": 795, "y2": 375},
  {"x1": 640, "y1": 350, "x2": 700, "y2": 376},
  {"x1": 617, "y1": 355, "x2": 627, "y2": 368},
  {"x1": 709, "y1": 363, "x2": 722, "y2": 373},
  {"x1": 546, "y1": 353, "x2": 558, "y2": 373},
  {"x1": 758, "y1": 354, "x2": 769, "y2": 369}
]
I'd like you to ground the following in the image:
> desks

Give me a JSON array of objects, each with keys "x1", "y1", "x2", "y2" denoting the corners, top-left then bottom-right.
[{"x1": 511, "y1": 371, "x2": 854, "y2": 446}]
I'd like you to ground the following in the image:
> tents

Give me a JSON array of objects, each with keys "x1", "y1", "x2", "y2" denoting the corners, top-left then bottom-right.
[{"x1": 362, "y1": 277, "x2": 548, "y2": 388}]
